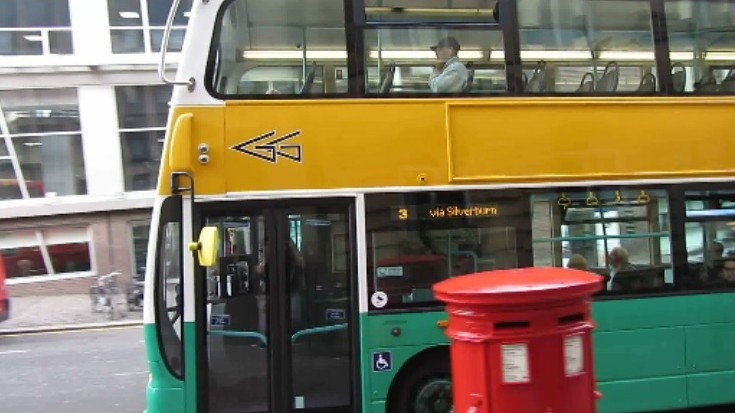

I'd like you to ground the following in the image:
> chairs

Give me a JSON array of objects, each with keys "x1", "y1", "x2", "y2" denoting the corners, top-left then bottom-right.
[
  {"x1": 599, "y1": 252, "x2": 735, "y2": 296},
  {"x1": 295, "y1": 59, "x2": 735, "y2": 95}
]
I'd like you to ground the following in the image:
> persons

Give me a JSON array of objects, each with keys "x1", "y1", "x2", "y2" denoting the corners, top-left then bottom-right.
[
  {"x1": 709, "y1": 242, "x2": 724, "y2": 265},
  {"x1": 454, "y1": 242, "x2": 493, "y2": 278},
  {"x1": 607, "y1": 246, "x2": 645, "y2": 295},
  {"x1": 252, "y1": 214, "x2": 306, "y2": 336},
  {"x1": 427, "y1": 37, "x2": 468, "y2": 94},
  {"x1": 566, "y1": 254, "x2": 589, "y2": 278},
  {"x1": 715, "y1": 257, "x2": 735, "y2": 291}
]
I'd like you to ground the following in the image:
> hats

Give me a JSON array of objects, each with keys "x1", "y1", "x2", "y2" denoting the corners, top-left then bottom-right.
[
  {"x1": 430, "y1": 37, "x2": 460, "y2": 51},
  {"x1": 567, "y1": 254, "x2": 587, "y2": 266}
]
